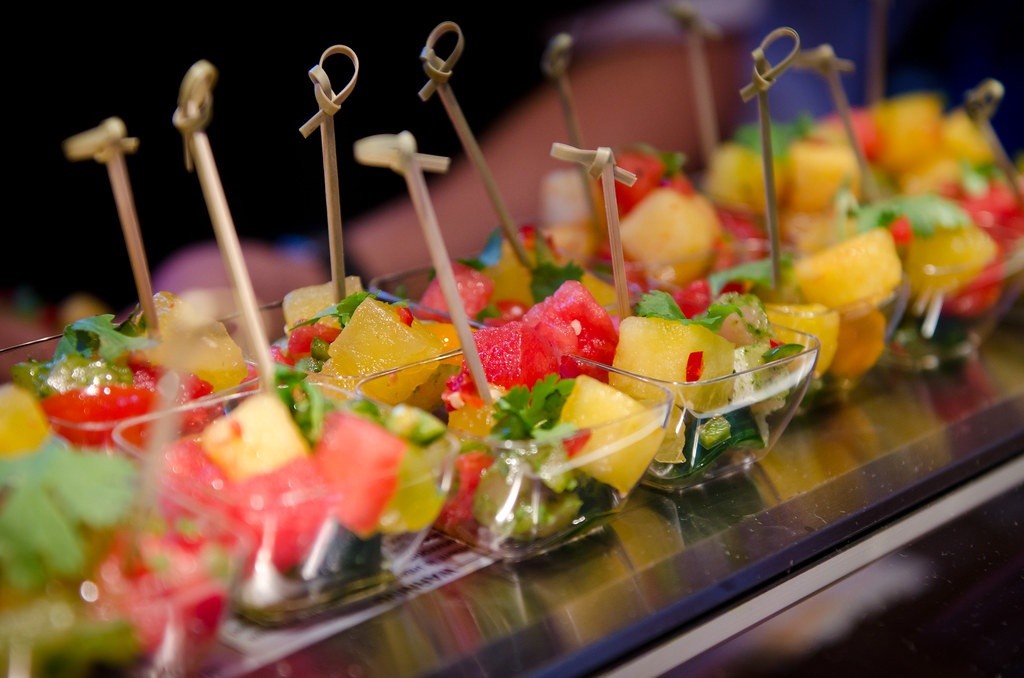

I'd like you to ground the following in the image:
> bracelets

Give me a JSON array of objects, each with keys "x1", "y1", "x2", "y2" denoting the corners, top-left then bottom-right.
[{"x1": 316, "y1": 233, "x2": 370, "y2": 291}]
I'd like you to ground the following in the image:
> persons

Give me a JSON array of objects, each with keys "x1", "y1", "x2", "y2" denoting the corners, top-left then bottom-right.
[{"x1": 0, "y1": 0, "x2": 756, "y2": 385}]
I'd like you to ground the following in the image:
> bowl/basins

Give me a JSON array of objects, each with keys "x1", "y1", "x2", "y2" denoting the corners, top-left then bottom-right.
[{"x1": 10, "y1": 2, "x2": 1021, "y2": 674}]
